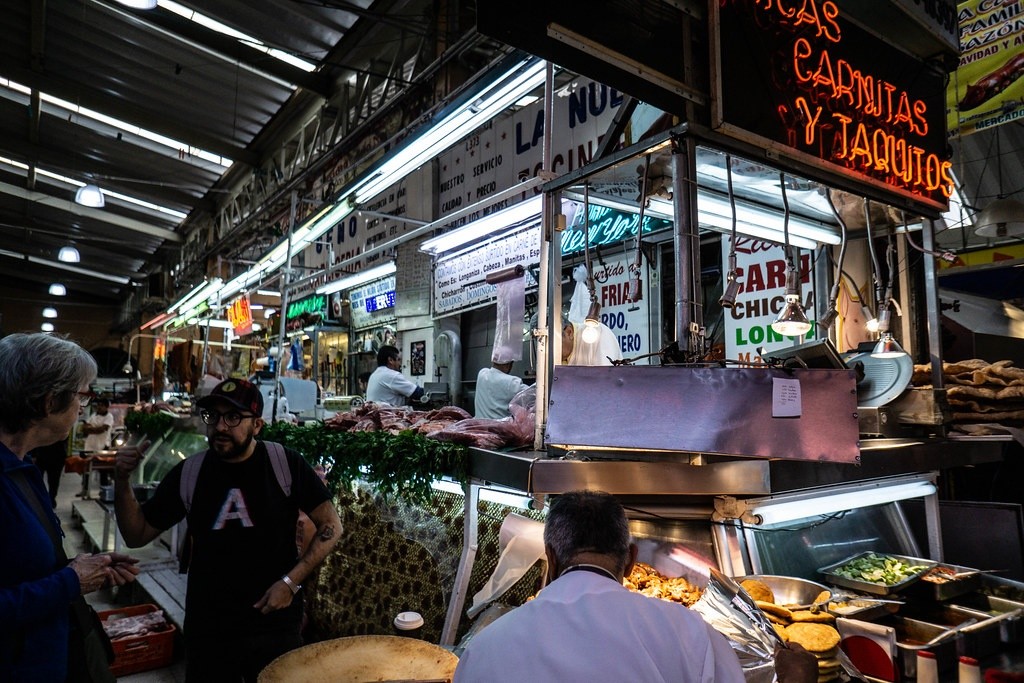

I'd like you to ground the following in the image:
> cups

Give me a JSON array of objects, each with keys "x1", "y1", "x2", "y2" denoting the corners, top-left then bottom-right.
[{"x1": 393, "y1": 612, "x2": 424, "y2": 639}]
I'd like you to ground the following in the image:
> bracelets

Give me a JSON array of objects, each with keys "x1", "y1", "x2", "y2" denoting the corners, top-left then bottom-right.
[{"x1": 281, "y1": 574, "x2": 300, "y2": 595}]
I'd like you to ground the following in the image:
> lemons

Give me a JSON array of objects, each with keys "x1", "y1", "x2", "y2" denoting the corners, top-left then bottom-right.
[{"x1": 832, "y1": 553, "x2": 928, "y2": 586}]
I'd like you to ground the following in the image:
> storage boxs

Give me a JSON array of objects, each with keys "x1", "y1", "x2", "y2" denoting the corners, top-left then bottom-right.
[{"x1": 96, "y1": 603, "x2": 177, "y2": 677}]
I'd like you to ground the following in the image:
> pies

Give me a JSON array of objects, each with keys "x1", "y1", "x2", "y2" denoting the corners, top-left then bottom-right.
[
  {"x1": 740, "y1": 579, "x2": 842, "y2": 683},
  {"x1": 911, "y1": 358, "x2": 1024, "y2": 436}
]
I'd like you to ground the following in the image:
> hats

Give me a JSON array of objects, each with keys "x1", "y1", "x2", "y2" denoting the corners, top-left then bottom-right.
[
  {"x1": 531, "y1": 303, "x2": 568, "y2": 339},
  {"x1": 197, "y1": 379, "x2": 265, "y2": 419},
  {"x1": 96, "y1": 396, "x2": 110, "y2": 407},
  {"x1": 493, "y1": 347, "x2": 521, "y2": 365}
]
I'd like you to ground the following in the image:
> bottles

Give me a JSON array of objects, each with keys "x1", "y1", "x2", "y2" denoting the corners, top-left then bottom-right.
[
  {"x1": 916, "y1": 650, "x2": 939, "y2": 683},
  {"x1": 958, "y1": 656, "x2": 981, "y2": 683}
]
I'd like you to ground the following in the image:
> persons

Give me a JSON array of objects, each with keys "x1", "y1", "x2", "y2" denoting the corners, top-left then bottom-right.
[
  {"x1": 365, "y1": 345, "x2": 424, "y2": 406},
  {"x1": 34, "y1": 435, "x2": 68, "y2": 508},
  {"x1": 75, "y1": 398, "x2": 114, "y2": 497},
  {"x1": 474, "y1": 361, "x2": 530, "y2": 418},
  {"x1": 113, "y1": 378, "x2": 344, "y2": 683},
  {"x1": 456, "y1": 491, "x2": 747, "y2": 683},
  {"x1": 0, "y1": 333, "x2": 140, "y2": 683},
  {"x1": 359, "y1": 371, "x2": 372, "y2": 393},
  {"x1": 530, "y1": 307, "x2": 623, "y2": 366}
]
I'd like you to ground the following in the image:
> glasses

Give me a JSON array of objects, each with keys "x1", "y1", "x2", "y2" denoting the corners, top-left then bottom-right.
[
  {"x1": 64, "y1": 387, "x2": 97, "y2": 407},
  {"x1": 201, "y1": 409, "x2": 255, "y2": 428}
]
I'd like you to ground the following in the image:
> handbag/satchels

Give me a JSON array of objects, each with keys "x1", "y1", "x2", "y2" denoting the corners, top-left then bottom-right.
[{"x1": 70, "y1": 603, "x2": 116, "y2": 683}]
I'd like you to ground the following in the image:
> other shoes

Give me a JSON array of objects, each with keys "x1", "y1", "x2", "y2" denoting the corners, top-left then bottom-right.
[{"x1": 75, "y1": 490, "x2": 90, "y2": 497}]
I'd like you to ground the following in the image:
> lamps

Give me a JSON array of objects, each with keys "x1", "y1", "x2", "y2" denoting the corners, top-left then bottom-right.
[
  {"x1": 57, "y1": 236, "x2": 81, "y2": 263},
  {"x1": 740, "y1": 480, "x2": 940, "y2": 528},
  {"x1": 123, "y1": 0, "x2": 1024, "y2": 372},
  {"x1": 40, "y1": 283, "x2": 67, "y2": 332},
  {"x1": 74, "y1": 173, "x2": 105, "y2": 208}
]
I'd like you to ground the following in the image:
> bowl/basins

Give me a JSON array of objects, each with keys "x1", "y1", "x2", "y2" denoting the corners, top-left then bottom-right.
[{"x1": 730, "y1": 575, "x2": 834, "y2": 611}]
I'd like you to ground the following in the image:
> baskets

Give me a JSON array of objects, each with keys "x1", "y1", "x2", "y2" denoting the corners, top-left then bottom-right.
[{"x1": 97, "y1": 605, "x2": 175, "y2": 677}]
